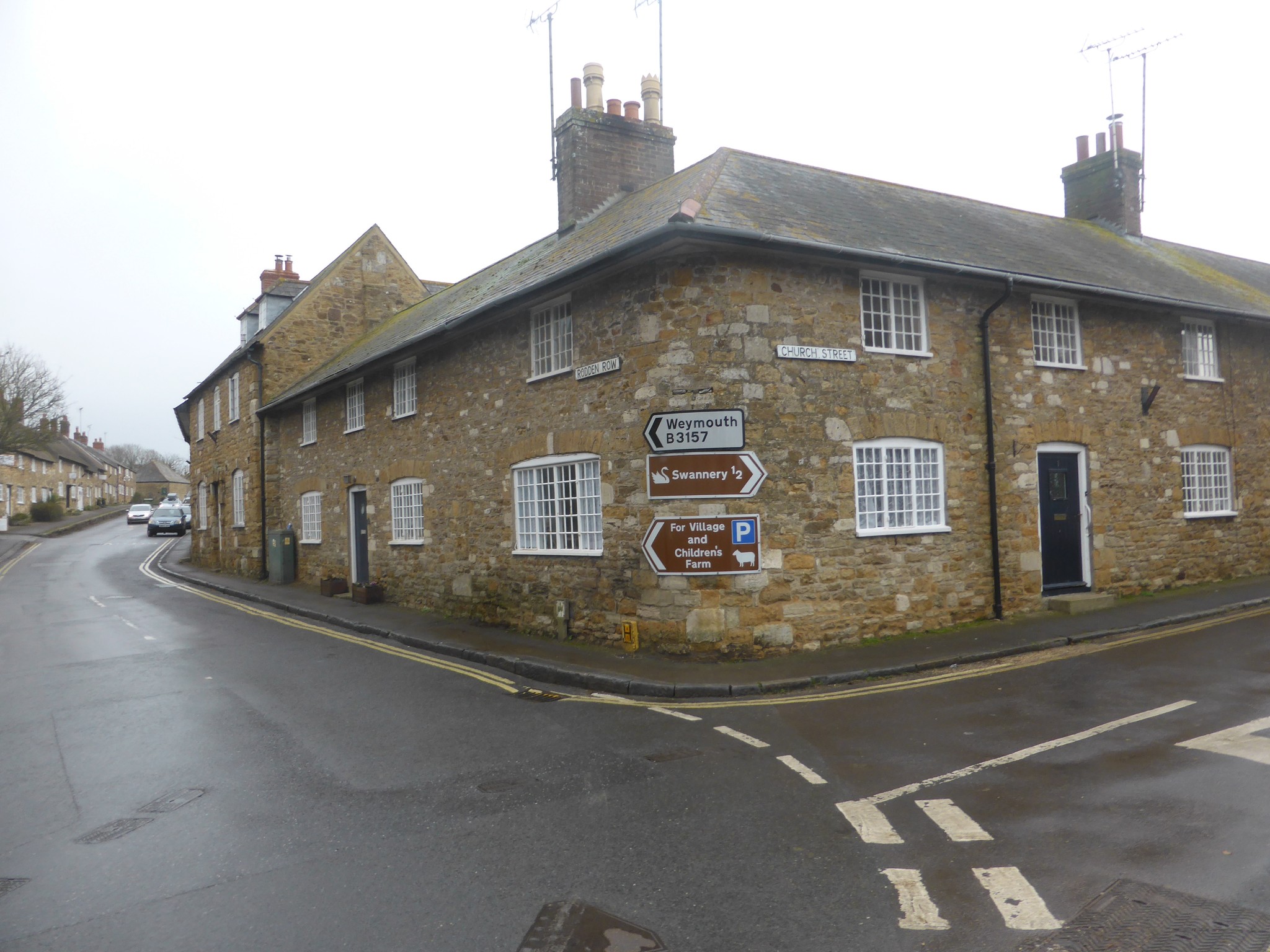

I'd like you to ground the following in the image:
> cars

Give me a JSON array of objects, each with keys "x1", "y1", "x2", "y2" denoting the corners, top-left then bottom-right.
[
  {"x1": 147, "y1": 506, "x2": 187, "y2": 537},
  {"x1": 182, "y1": 497, "x2": 191, "y2": 504},
  {"x1": 172, "y1": 503, "x2": 192, "y2": 529},
  {"x1": 159, "y1": 496, "x2": 182, "y2": 508},
  {"x1": 126, "y1": 503, "x2": 155, "y2": 525}
]
